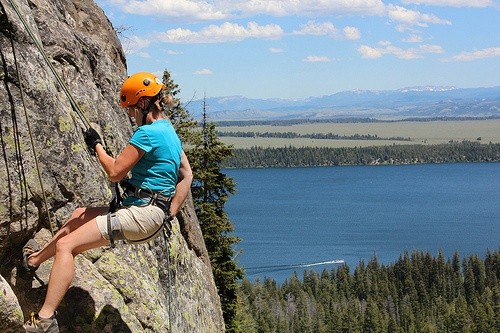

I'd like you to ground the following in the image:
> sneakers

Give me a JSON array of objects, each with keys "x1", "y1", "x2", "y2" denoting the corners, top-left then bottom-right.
[
  {"x1": 19, "y1": 242, "x2": 40, "y2": 279},
  {"x1": 15, "y1": 311, "x2": 60, "y2": 333}
]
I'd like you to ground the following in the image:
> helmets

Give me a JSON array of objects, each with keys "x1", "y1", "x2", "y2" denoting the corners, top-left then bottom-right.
[{"x1": 120, "y1": 72, "x2": 165, "y2": 108}]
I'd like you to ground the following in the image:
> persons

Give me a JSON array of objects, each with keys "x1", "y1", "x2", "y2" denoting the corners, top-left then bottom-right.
[{"x1": 12, "y1": 72, "x2": 193, "y2": 333}]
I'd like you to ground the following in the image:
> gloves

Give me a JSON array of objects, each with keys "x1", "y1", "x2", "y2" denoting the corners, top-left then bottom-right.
[{"x1": 85, "y1": 128, "x2": 101, "y2": 147}]
